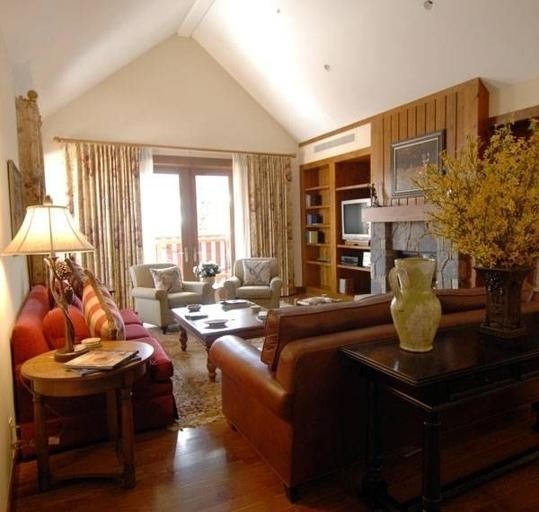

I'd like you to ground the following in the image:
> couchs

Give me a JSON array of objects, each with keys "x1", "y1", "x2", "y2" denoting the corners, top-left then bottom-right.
[
  {"x1": 11, "y1": 283, "x2": 179, "y2": 463},
  {"x1": 209, "y1": 285, "x2": 538, "y2": 503}
]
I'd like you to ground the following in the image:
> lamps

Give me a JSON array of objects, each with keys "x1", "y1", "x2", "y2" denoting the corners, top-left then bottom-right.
[{"x1": 0, "y1": 196, "x2": 98, "y2": 363}]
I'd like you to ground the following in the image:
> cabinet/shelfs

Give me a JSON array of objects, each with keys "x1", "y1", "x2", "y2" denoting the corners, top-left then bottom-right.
[{"x1": 299, "y1": 145, "x2": 373, "y2": 301}]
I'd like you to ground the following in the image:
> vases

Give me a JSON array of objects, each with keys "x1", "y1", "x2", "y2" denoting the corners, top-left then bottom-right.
[{"x1": 388, "y1": 256, "x2": 441, "y2": 354}]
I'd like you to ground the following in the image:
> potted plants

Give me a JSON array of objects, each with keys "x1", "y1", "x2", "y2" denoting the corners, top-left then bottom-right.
[
  {"x1": 404, "y1": 110, "x2": 539, "y2": 340},
  {"x1": 193, "y1": 260, "x2": 222, "y2": 285}
]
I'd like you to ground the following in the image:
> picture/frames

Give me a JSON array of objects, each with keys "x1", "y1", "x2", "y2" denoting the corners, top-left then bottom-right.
[
  {"x1": 390, "y1": 128, "x2": 447, "y2": 200},
  {"x1": 7, "y1": 160, "x2": 25, "y2": 241}
]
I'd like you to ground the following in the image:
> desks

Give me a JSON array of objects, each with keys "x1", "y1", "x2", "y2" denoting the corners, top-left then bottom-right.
[
  {"x1": 19, "y1": 340, "x2": 155, "y2": 490},
  {"x1": 336, "y1": 321, "x2": 539, "y2": 511}
]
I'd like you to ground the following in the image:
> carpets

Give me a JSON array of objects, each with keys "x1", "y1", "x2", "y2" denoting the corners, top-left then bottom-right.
[{"x1": 146, "y1": 326, "x2": 267, "y2": 433}]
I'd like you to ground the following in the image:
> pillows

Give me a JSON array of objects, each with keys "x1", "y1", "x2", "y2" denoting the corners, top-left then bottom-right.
[
  {"x1": 43, "y1": 304, "x2": 92, "y2": 350},
  {"x1": 149, "y1": 265, "x2": 184, "y2": 293},
  {"x1": 82, "y1": 268, "x2": 127, "y2": 341},
  {"x1": 241, "y1": 259, "x2": 274, "y2": 285}
]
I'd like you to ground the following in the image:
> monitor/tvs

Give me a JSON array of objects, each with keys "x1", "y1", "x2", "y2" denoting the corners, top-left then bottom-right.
[{"x1": 340, "y1": 198, "x2": 373, "y2": 245}]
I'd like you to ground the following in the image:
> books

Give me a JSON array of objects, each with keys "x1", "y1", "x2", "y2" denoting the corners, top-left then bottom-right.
[{"x1": 63, "y1": 350, "x2": 141, "y2": 377}]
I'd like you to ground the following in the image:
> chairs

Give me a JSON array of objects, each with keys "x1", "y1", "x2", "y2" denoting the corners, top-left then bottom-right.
[
  {"x1": 223, "y1": 257, "x2": 283, "y2": 308},
  {"x1": 128, "y1": 263, "x2": 216, "y2": 335}
]
